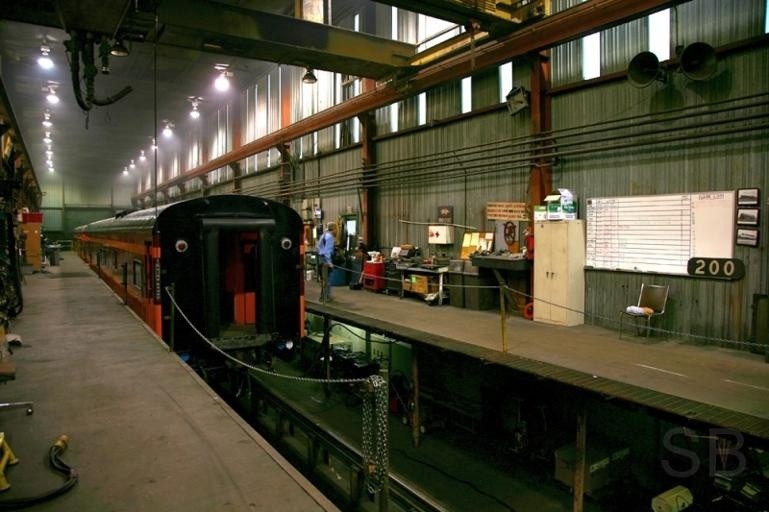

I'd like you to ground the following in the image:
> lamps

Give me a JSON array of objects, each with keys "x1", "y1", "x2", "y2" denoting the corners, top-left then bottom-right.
[
  {"x1": 122, "y1": 65, "x2": 234, "y2": 177},
  {"x1": 110, "y1": 37, "x2": 129, "y2": 57},
  {"x1": 36, "y1": 43, "x2": 59, "y2": 172},
  {"x1": 302, "y1": 65, "x2": 317, "y2": 83}
]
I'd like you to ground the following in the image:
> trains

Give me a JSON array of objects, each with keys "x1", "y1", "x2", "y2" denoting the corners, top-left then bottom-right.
[{"x1": 71, "y1": 194, "x2": 306, "y2": 363}]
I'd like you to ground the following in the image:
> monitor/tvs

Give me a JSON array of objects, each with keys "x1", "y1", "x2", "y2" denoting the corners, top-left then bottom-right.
[{"x1": 346, "y1": 219, "x2": 357, "y2": 236}]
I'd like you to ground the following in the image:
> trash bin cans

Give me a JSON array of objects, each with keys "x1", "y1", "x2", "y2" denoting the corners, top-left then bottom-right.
[
  {"x1": 363, "y1": 261, "x2": 384, "y2": 292},
  {"x1": 47, "y1": 244, "x2": 62, "y2": 266}
]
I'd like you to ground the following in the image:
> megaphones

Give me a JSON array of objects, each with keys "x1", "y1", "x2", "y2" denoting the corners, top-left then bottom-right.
[
  {"x1": 626, "y1": 51, "x2": 666, "y2": 88},
  {"x1": 679, "y1": 42, "x2": 717, "y2": 80}
]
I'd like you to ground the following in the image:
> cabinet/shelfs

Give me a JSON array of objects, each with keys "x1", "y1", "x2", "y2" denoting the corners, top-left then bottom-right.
[{"x1": 534, "y1": 220, "x2": 585, "y2": 327}]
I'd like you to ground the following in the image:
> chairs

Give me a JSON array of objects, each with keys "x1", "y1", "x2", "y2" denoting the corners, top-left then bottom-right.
[{"x1": 619, "y1": 282, "x2": 671, "y2": 343}]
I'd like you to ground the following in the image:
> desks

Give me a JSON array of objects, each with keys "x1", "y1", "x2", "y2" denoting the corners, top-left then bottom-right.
[{"x1": 396, "y1": 264, "x2": 449, "y2": 306}]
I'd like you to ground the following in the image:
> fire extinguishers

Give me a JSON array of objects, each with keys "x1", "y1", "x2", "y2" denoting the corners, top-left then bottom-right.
[{"x1": 522, "y1": 227, "x2": 534, "y2": 260}]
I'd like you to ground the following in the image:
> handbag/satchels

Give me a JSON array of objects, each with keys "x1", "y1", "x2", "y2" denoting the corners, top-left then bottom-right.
[{"x1": 331, "y1": 247, "x2": 346, "y2": 263}]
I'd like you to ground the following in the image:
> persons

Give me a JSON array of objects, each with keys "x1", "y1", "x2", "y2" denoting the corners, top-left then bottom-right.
[{"x1": 314, "y1": 220, "x2": 365, "y2": 292}]
